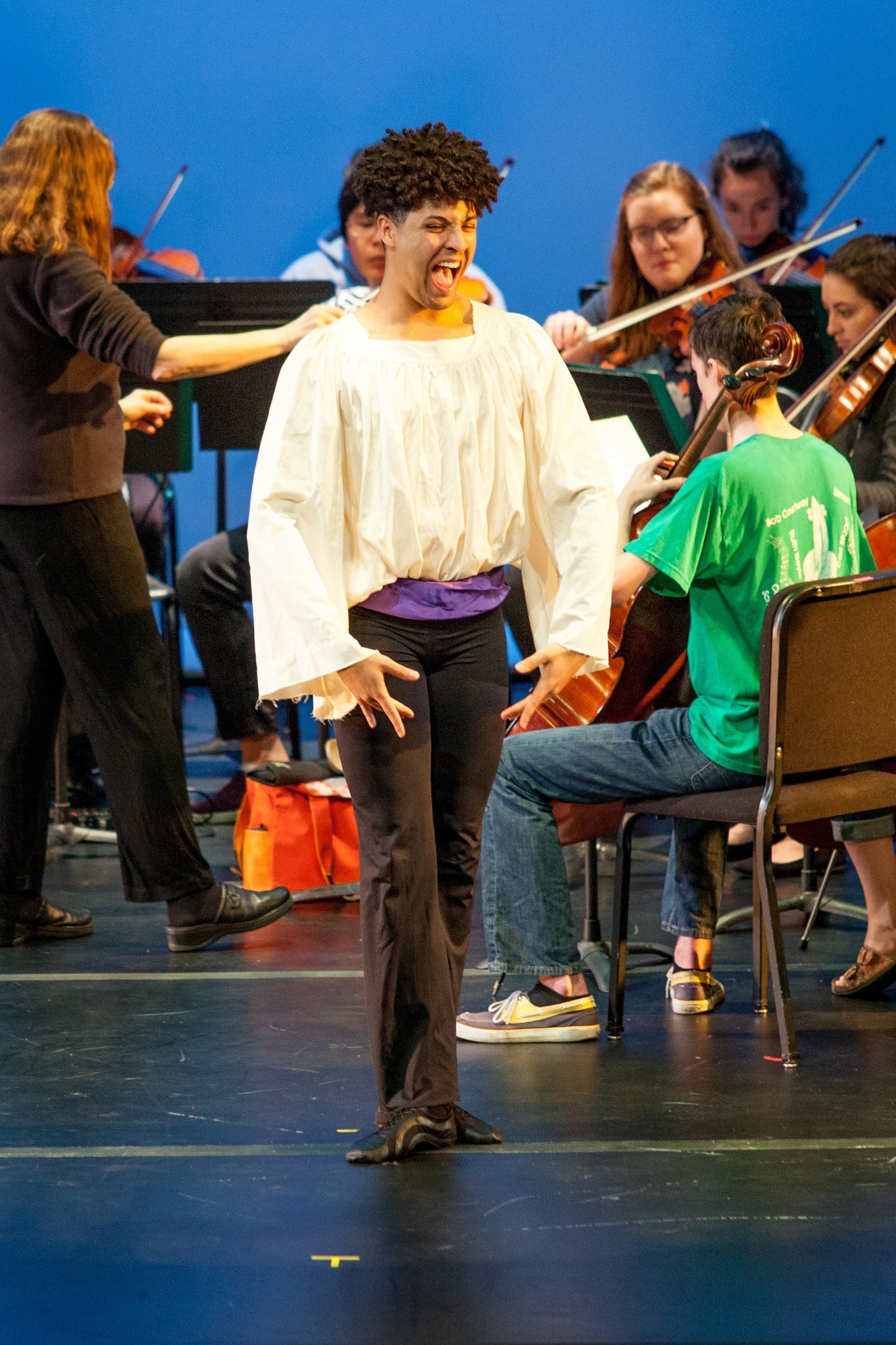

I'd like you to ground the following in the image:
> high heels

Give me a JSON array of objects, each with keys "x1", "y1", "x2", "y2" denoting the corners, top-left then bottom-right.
[
  {"x1": 165, "y1": 884, "x2": 292, "y2": 951},
  {"x1": 0, "y1": 899, "x2": 92, "y2": 945}
]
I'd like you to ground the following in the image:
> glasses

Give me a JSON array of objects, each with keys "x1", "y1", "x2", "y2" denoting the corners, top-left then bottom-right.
[{"x1": 627, "y1": 211, "x2": 699, "y2": 246}]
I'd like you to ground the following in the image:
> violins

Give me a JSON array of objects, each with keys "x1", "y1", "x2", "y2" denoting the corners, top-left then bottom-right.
[
  {"x1": 800, "y1": 336, "x2": 896, "y2": 441},
  {"x1": 648, "y1": 261, "x2": 737, "y2": 355},
  {"x1": 109, "y1": 225, "x2": 206, "y2": 281},
  {"x1": 743, "y1": 231, "x2": 831, "y2": 288}
]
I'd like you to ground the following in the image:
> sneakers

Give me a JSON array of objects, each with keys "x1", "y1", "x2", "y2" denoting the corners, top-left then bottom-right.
[
  {"x1": 453, "y1": 981, "x2": 600, "y2": 1043},
  {"x1": 663, "y1": 963, "x2": 725, "y2": 1014}
]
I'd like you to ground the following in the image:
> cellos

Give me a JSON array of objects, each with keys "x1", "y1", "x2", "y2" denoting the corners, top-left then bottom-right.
[{"x1": 490, "y1": 320, "x2": 804, "y2": 998}]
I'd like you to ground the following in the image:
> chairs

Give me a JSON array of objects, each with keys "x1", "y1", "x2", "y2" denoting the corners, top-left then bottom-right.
[{"x1": 579, "y1": 567, "x2": 896, "y2": 1070}]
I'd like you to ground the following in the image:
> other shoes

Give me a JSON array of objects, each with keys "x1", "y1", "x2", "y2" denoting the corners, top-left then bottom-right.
[
  {"x1": 192, "y1": 770, "x2": 250, "y2": 824},
  {"x1": 734, "y1": 848, "x2": 846, "y2": 877},
  {"x1": 722, "y1": 838, "x2": 757, "y2": 859}
]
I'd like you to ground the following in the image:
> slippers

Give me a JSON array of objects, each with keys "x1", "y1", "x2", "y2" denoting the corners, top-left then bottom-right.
[{"x1": 832, "y1": 945, "x2": 896, "y2": 995}]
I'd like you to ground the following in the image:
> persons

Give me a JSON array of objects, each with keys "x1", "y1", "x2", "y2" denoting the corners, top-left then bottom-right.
[
  {"x1": 457, "y1": 294, "x2": 876, "y2": 1043},
  {"x1": 1, "y1": 109, "x2": 336, "y2": 950},
  {"x1": 711, "y1": 130, "x2": 831, "y2": 283},
  {"x1": 108, "y1": 226, "x2": 165, "y2": 578},
  {"x1": 540, "y1": 162, "x2": 847, "y2": 879},
  {"x1": 283, "y1": 148, "x2": 509, "y2": 312},
  {"x1": 249, "y1": 122, "x2": 618, "y2": 1164},
  {"x1": 822, "y1": 233, "x2": 896, "y2": 996},
  {"x1": 175, "y1": 169, "x2": 487, "y2": 824}
]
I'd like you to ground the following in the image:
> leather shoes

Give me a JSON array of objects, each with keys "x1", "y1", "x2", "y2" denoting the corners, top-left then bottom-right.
[
  {"x1": 345, "y1": 1110, "x2": 456, "y2": 1162},
  {"x1": 452, "y1": 1105, "x2": 501, "y2": 1143}
]
